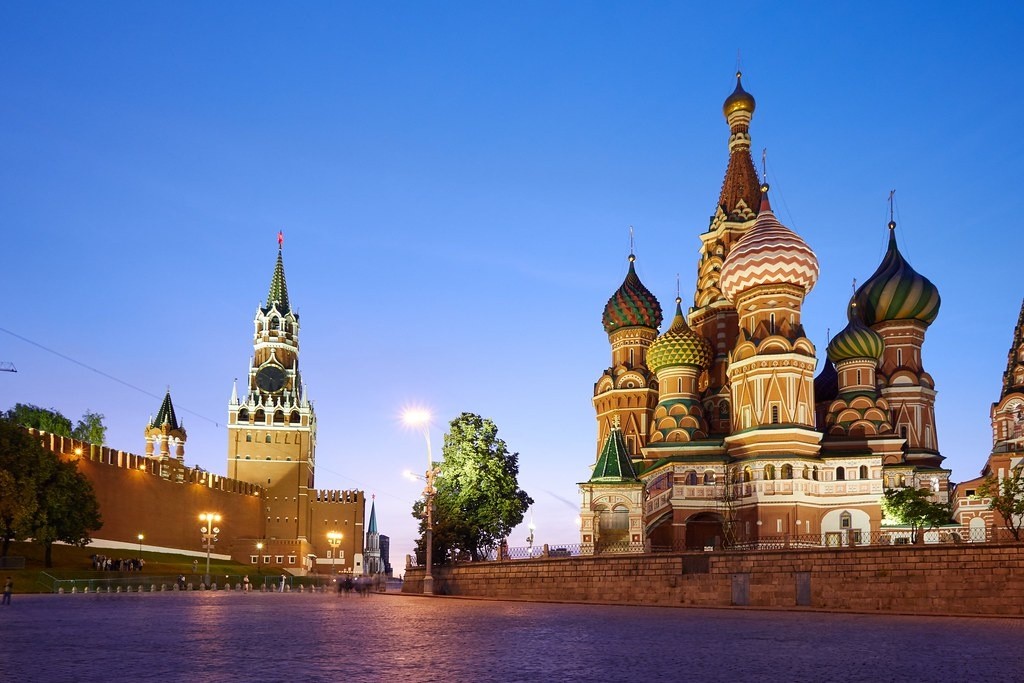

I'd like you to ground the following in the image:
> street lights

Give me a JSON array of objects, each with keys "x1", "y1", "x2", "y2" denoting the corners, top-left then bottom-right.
[
  {"x1": 138, "y1": 528, "x2": 144, "y2": 560},
  {"x1": 199, "y1": 510, "x2": 221, "y2": 589},
  {"x1": 401, "y1": 404, "x2": 435, "y2": 595},
  {"x1": 256, "y1": 542, "x2": 263, "y2": 573},
  {"x1": 326, "y1": 530, "x2": 342, "y2": 576}
]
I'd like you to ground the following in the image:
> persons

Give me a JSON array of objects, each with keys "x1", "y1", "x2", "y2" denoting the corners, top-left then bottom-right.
[
  {"x1": 0, "y1": 576, "x2": 13, "y2": 605},
  {"x1": 336, "y1": 571, "x2": 386, "y2": 599},
  {"x1": 194, "y1": 465, "x2": 200, "y2": 470},
  {"x1": 92, "y1": 554, "x2": 145, "y2": 572},
  {"x1": 243, "y1": 574, "x2": 250, "y2": 595},
  {"x1": 177, "y1": 574, "x2": 186, "y2": 586},
  {"x1": 193, "y1": 559, "x2": 198, "y2": 571},
  {"x1": 278, "y1": 572, "x2": 286, "y2": 593}
]
